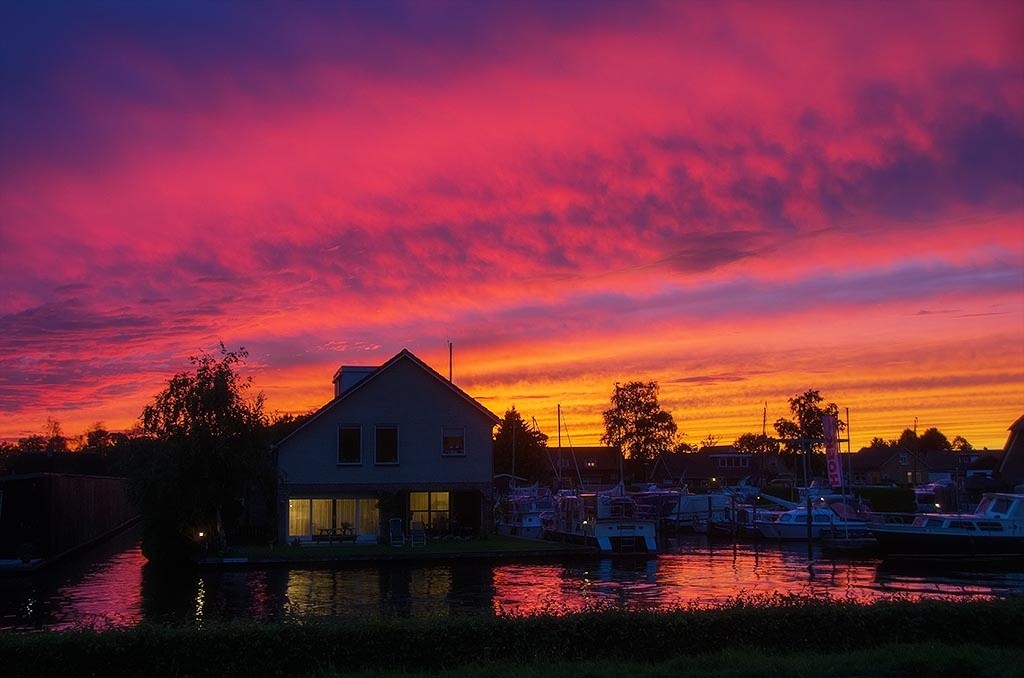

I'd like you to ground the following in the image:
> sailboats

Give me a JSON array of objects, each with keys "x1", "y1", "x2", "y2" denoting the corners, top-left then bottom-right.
[{"x1": 496, "y1": 382, "x2": 683, "y2": 555}]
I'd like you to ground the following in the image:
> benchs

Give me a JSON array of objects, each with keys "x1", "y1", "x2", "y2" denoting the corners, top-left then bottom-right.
[{"x1": 317, "y1": 535, "x2": 360, "y2": 539}]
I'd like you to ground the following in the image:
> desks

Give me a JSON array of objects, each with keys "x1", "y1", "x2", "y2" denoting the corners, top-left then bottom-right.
[{"x1": 316, "y1": 527, "x2": 355, "y2": 545}]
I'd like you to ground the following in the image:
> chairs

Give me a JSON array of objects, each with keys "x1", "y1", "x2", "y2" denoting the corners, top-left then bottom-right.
[
  {"x1": 410, "y1": 519, "x2": 427, "y2": 548},
  {"x1": 389, "y1": 518, "x2": 405, "y2": 548}
]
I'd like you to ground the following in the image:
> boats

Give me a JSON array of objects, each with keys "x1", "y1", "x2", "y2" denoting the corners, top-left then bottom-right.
[{"x1": 680, "y1": 470, "x2": 1024, "y2": 563}]
